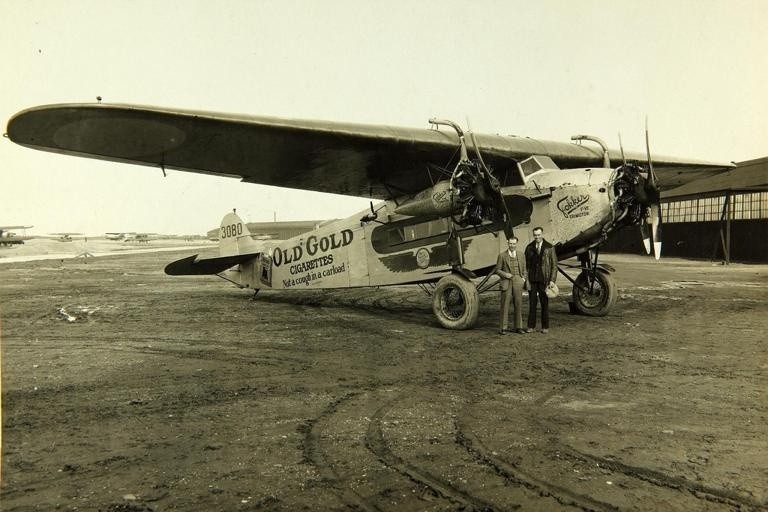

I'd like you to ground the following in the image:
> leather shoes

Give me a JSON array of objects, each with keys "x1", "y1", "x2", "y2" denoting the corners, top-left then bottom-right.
[
  {"x1": 526, "y1": 328, "x2": 537, "y2": 332},
  {"x1": 516, "y1": 328, "x2": 525, "y2": 334},
  {"x1": 501, "y1": 330, "x2": 507, "y2": 335},
  {"x1": 542, "y1": 328, "x2": 549, "y2": 334}
]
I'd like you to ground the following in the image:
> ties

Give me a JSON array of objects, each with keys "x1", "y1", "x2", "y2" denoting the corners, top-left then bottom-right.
[{"x1": 537, "y1": 243, "x2": 540, "y2": 255}]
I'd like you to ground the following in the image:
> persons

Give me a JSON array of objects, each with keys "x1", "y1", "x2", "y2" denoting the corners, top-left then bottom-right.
[
  {"x1": 495, "y1": 235, "x2": 527, "y2": 335},
  {"x1": 522, "y1": 226, "x2": 559, "y2": 334}
]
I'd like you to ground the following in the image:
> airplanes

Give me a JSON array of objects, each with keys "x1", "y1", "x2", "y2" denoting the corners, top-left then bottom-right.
[
  {"x1": 3, "y1": 96, "x2": 738, "y2": 333},
  {"x1": 0, "y1": 226, "x2": 159, "y2": 247}
]
